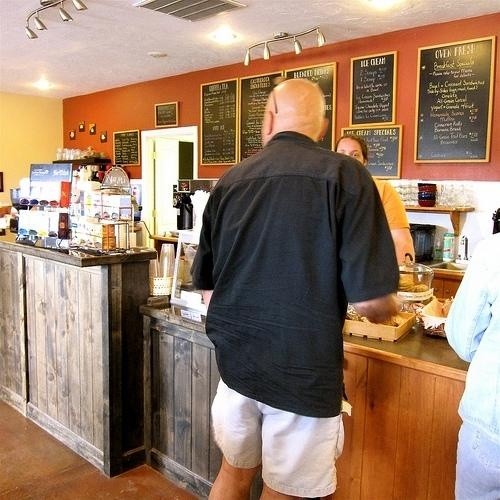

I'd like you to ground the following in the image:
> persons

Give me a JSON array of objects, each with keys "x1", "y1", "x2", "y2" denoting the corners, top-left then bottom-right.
[
  {"x1": 332, "y1": 135, "x2": 417, "y2": 266},
  {"x1": 188, "y1": 76, "x2": 399, "y2": 500},
  {"x1": 443, "y1": 230, "x2": 499, "y2": 500}
]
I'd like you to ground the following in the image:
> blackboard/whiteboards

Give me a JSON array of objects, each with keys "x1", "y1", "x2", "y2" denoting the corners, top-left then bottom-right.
[
  {"x1": 414, "y1": 35, "x2": 496, "y2": 164},
  {"x1": 154, "y1": 101, "x2": 179, "y2": 128},
  {"x1": 200, "y1": 62, "x2": 338, "y2": 166},
  {"x1": 341, "y1": 50, "x2": 404, "y2": 180},
  {"x1": 113, "y1": 129, "x2": 141, "y2": 167}
]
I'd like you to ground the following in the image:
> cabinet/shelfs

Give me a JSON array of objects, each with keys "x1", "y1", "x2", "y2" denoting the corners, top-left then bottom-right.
[
  {"x1": 428, "y1": 270, "x2": 466, "y2": 303},
  {"x1": 153, "y1": 236, "x2": 200, "y2": 260}
]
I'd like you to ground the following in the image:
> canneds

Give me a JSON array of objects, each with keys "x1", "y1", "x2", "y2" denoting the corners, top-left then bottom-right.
[
  {"x1": 102, "y1": 224, "x2": 115, "y2": 237},
  {"x1": 102, "y1": 237, "x2": 116, "y2": 251}
]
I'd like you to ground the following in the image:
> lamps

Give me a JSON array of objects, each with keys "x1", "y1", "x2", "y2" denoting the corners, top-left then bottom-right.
[
  {"x1": 244, "y1": 24, "x2": 324, "y2": 66},
  {"x1": 23, "y1": 0, "x2": 88, "y2": 38}
]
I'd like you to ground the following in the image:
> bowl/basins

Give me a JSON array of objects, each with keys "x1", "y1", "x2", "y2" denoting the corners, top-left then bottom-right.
[{"x1": 417, "y1": 182, "x2": 437, "y2": 208}]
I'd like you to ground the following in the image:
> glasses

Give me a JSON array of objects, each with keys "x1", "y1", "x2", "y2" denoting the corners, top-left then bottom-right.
[{"x1": 270, "y1": 76, "x2": 320, "y2": 116}]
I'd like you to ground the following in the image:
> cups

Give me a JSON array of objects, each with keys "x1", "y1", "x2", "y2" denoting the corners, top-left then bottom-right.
[{"x1": 158, "y1": 242, "x2": 177, "y2": 279}]
